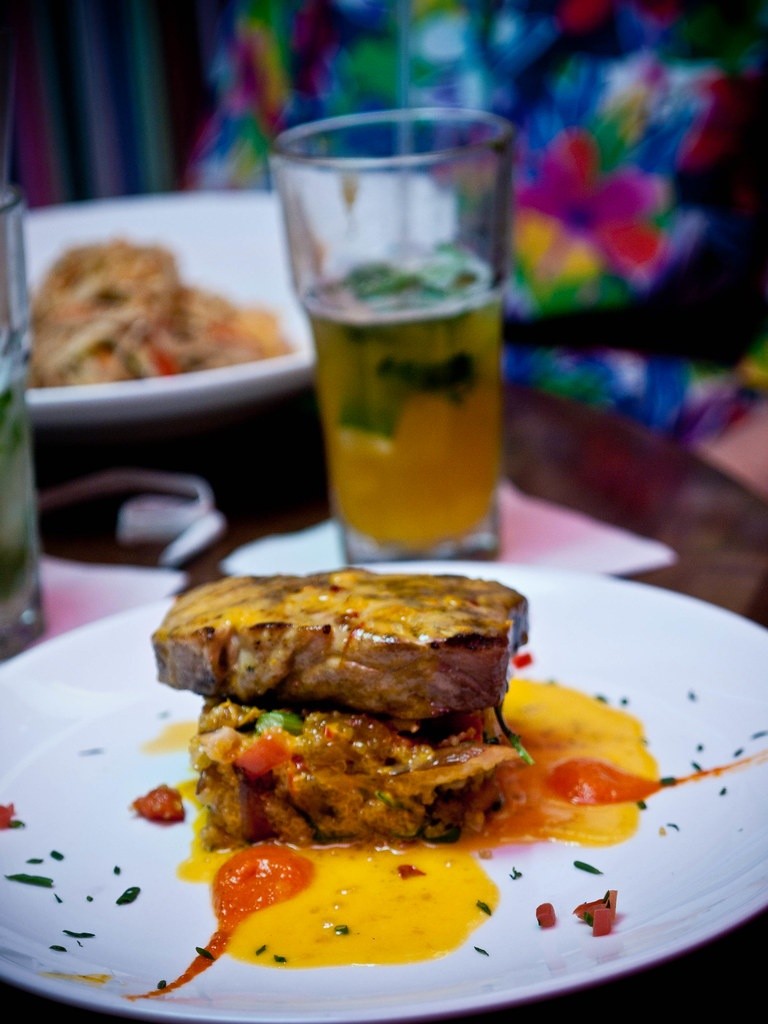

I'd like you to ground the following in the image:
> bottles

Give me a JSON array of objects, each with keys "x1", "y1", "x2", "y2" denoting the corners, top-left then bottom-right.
[{"x1": 0, "y1": 150, "x2": 46, "y2": 662}]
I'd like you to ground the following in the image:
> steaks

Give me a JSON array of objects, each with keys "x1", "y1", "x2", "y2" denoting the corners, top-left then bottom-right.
[{"x1": 149, "y1": 566, "x2": 529, "y2": 716}]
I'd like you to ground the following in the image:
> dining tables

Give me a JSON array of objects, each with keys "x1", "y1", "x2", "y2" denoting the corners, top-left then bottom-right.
[{"x1": 3, "y1": 375, "x2": 768, "y2": 1024}]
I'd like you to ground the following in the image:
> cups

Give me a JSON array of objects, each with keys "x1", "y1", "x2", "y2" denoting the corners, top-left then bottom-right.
[{"x1": 269, "y1": 106, "x2": 510, "y2": 562}]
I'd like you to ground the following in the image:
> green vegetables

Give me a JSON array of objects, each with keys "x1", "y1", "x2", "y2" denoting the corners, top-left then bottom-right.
[{"x1": 340, "y1": 253, "x2": 493, "y2": 444}]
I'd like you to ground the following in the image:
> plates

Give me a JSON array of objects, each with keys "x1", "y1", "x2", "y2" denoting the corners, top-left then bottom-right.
[
  {"x1": 22, "y1": 189, "x2": 316, "y2": 423},
  {"x1": 0, "y1": 560, "x2": 768, "y2": 1022}
]
